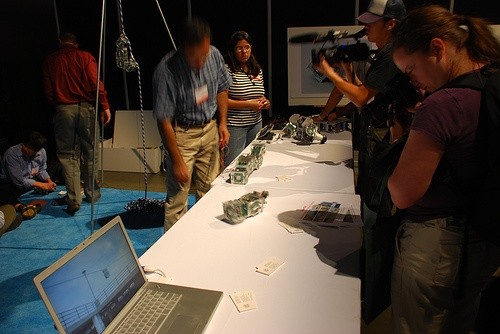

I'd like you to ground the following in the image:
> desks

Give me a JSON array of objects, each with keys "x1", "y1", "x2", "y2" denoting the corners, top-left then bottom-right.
[
  {"x1": 138, "y1": 185, "x2": 363, "y2": 334},
  {"x1": 210, "y1": 129, "x2": 356, "y2": 194}
]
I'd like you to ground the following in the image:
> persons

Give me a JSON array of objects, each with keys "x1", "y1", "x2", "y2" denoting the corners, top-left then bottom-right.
[
  {"x1": 44, "y1": 31, "x2": 111, "y2": 213},
  {"x1": 314, "y1": 46, "x2": 375, "y2": 123},
  {"x1": 0, "y1": 132, "x2": 57, "y2": 197},
  {"x1": 223, "y1": 31, "x2": 271, "y2": 167},
  {"x1": 387, "y1": 6, "x2": 500, "y2": 334},
  {"x1": 389, "y1": 92, "x2": 475, "y2": 334},
  {"x1": 153, "y1": 21, "x2": 231, "y2": 233},
  {"x1": 317, "y1": 0, "x2": 421, "y2": 190}
]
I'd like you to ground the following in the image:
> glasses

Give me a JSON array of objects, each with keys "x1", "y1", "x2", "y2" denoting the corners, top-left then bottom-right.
[
  {"x1": 234, "y1": 45, "x2": 252, "y2": 52},
  {"x1": 404, "y1": 47, "x2": 430, "y2": 76}
]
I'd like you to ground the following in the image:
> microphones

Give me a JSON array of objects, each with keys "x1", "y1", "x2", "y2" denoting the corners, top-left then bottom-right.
[{"x1": 288, "y1": 32, "x2": 319, "y2": 45}]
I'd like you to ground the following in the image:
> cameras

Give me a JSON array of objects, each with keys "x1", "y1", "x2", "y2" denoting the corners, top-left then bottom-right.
[{"x1": 361, "y1": 74, "x2": 408, "y2": 129}]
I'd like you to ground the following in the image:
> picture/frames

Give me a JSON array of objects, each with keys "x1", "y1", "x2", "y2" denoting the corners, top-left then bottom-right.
[{"x1": 288, "y1": 26, "x2": 377, "y2": 107}]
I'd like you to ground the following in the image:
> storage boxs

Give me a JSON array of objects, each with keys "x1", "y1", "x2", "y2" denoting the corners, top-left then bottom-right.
[{"x1": 100, "y1": 138, "x2": 162, "y2": 173}]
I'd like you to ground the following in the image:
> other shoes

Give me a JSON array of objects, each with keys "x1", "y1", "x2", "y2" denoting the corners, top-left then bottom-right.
[
  {"x1": 14, "y1": 202, "x2": 40, "y2": 221},
  {"x1": 66, "y1": 204, "x2": 81, "y2": 214},
  {"x1": 88, "y1": 194, "x2": 102, "y2": 203},
  {"x1": 0, "y1": 203, "x2": 23, "y2": 236}
]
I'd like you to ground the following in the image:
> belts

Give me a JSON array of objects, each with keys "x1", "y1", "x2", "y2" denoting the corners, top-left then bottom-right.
[{"x1": 172, "y1": 119, "x2": 212, "y2": 128}]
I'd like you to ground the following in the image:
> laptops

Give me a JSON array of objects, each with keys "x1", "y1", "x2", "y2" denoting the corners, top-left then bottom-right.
[{"x1": 33, "y1": 215, "x2": 224, "y2": 334}]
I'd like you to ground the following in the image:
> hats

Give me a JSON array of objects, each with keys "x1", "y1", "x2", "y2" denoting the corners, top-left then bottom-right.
[{"x1": 355, "y1": 0, "x2": 407, "y2": 23}]
